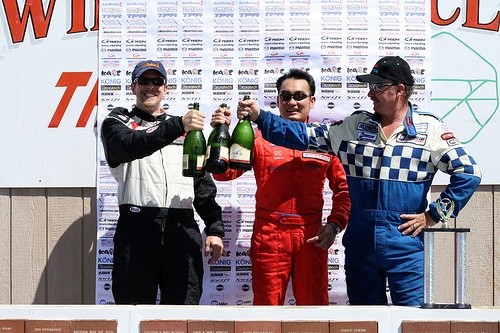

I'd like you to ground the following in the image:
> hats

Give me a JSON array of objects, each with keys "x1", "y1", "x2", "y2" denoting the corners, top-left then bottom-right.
[
  {"x1": 356, "y1": 56, "x2": 413, "y2": 86},
  {"x1": 131, "y1": 58, "x2": 167, "y2": 84}
]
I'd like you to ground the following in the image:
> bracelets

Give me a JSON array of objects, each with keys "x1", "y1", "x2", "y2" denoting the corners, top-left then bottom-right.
[{"x1": 329, "y1": 223, "x2": 341, "y2": 234}]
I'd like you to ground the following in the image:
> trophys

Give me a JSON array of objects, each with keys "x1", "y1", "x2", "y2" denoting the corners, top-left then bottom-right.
[{"x1": 419, "y1": 198, "x2": 472, "y2": 309}]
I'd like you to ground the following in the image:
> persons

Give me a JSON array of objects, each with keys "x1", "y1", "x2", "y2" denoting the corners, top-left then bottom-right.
[
  {"x1": 101, "y1": 60, "x2": 225, "y2": 305},
  {"x1": 211, "y1": 69, "x2": 351, "y2": 306},
  {"x1": 236, "y1": 56, "x2": 482, "y2": 306}
]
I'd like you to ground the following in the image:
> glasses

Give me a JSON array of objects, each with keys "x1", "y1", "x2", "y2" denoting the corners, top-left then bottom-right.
[
  {"x1": 136, "y1": 77, "x2": 164, "y2": 86},
  {"x1": 369, "y1": 82, "x2": 396, "y2": 93},
  {"x1": 279, "y1": 93, "x2": 310, "y2": 101}
]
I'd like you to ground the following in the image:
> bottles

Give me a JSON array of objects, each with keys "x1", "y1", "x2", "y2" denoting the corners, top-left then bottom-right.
[
  {"x1": 182, "y1": 102, "x2": 206, "y2": 177},
  {"x1": 204, "y1": 103, "x2": 231, "y2": 174},
  {"x1": 229, "y1": 95, "x2": 254, "y2": 171}
]
